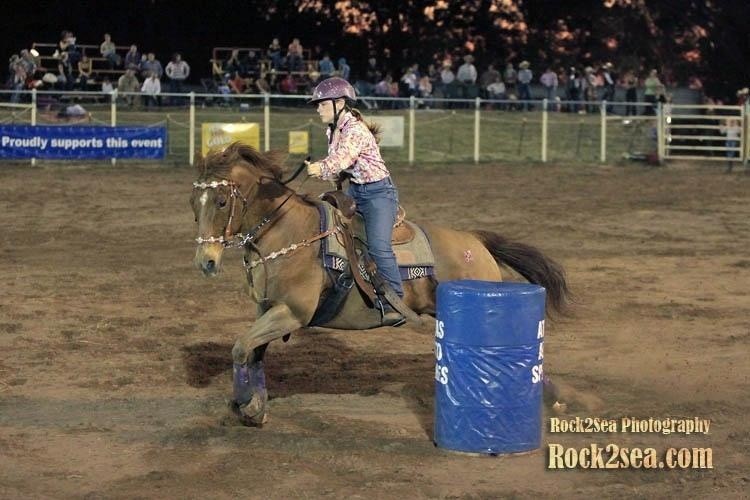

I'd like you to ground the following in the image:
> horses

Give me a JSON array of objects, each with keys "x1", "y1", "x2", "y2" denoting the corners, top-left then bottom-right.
[{"x1": 189, "y1": 140, "x2": 593, "y2": 428}]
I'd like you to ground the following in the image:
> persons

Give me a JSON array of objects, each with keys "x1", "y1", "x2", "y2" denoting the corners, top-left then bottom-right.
[
  {"x1": 9, "y1": 31, "x2": 750, "y2": 176},
  {"x1": 307, "y1": 76, "x2": 404, "y2": 326}
]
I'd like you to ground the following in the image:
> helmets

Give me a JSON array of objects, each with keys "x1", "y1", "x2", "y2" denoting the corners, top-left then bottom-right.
[{"x1": 306, "y1": 76, "x2": 356, "y2": 104}]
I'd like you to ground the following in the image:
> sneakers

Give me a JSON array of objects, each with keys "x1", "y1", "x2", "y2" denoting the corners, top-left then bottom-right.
[{"x1": 381, "y1": 312, "x2": 402, "y2": 324}]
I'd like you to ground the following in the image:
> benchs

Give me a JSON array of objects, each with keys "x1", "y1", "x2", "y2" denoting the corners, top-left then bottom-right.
[
  {"x1": 30, "y1": 42, "x2": 137, "y2": 85},
  {"x1": 210, "y1": 46, "x2": 350, "y2": 85}
]
